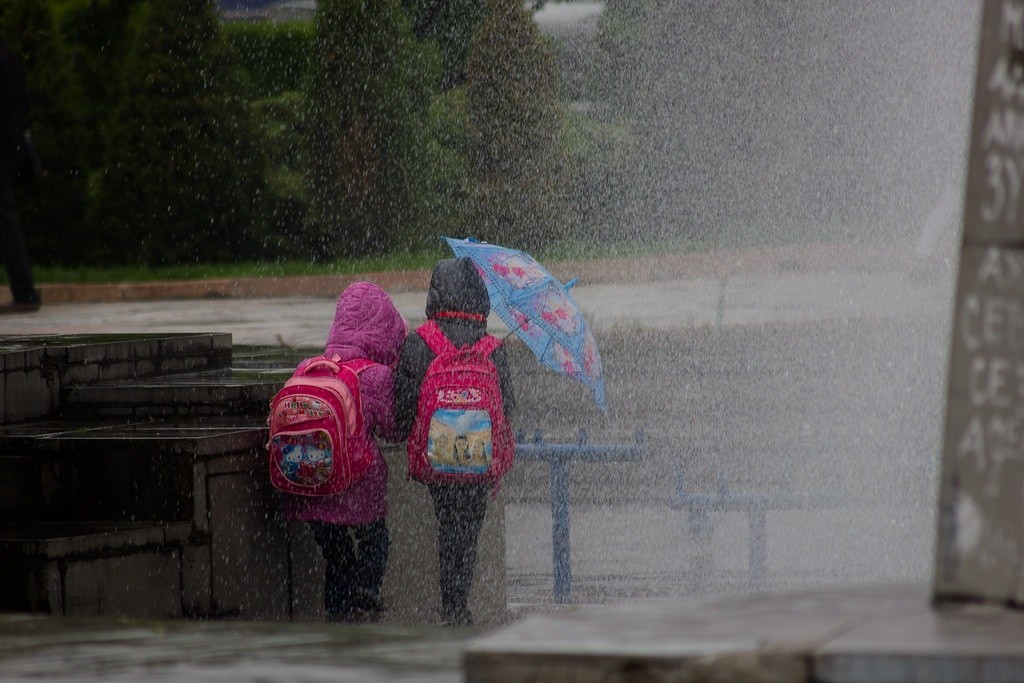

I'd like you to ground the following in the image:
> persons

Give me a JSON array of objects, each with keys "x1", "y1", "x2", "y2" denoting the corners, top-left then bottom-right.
[
  {"x1": 291, "y1": 281, "x2": 407, "y2": 623},
  {"x1": 0, "y1": 134, "x2": 42, "y2": 308},
  {"x1": 391, "y1": 258, "x2": 517, "y2": 625}
]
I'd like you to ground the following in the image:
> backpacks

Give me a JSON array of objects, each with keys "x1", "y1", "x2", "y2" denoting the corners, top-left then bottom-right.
[
  {"x1": 408, "y1": 325, "x2": 514, "y2": 485},
  {"x1": 269, "y1": 356, "x2": 376, "y2": 497}
]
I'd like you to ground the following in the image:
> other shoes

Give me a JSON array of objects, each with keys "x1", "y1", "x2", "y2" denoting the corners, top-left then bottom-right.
[
  {"x1": 353, "y1": 603, "x2": 386, "y2": 621},
  {"x1": 23, "y1": 287, "x2": 43, "y2": 311}
]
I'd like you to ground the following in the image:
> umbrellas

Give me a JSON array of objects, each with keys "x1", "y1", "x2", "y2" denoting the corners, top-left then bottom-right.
[{"x1": 443, "y1": 237, "x2": 607, "y2": 408}]
